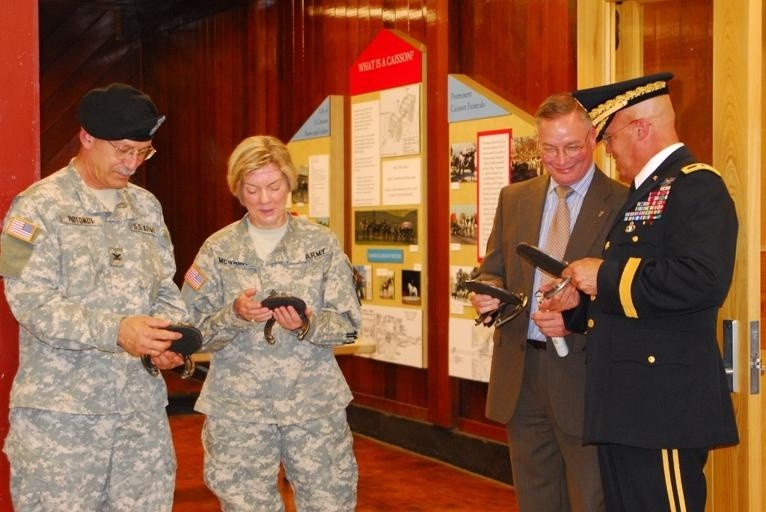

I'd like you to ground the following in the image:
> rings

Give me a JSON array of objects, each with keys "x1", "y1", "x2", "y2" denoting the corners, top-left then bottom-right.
[{"x1": 249, "y1": 317, "x2": 257, "y2": 323}]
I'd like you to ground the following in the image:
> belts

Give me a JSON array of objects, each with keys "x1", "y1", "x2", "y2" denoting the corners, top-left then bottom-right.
[{"x1": 526, "y1": 337, "x2": 547, "y2": 350}]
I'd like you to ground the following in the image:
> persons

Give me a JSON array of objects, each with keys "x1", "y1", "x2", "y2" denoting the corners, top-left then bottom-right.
[
  {"x1": 0, "y1": 80, "x2": 190, "y2": 512},
  {"x1": 355, "y1": 215, "x2": 417, "y2": 243},
  {"x1": 452, "y1": 149, "x2": 478, "y2": 178},
  {"x1": 179, "y1": 133, "x2": 364, "y2": 512},
  {"x1": 537, "y1": 70, "x2": 742, "y2": 512},
  {"x1": 452, "y1": 266, "x2": 479, "y2": 299},
  {"x1": 466, "y1": 93, "x2": 634, "y2": 511}
]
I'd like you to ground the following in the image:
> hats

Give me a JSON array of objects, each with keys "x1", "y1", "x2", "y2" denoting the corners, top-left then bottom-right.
[
  {"x1": 572, "y1": 72, "x2": 673, "y2": 143},
  {"x1": 77, "y1": 84, "x2": 166, "y2": 141}
]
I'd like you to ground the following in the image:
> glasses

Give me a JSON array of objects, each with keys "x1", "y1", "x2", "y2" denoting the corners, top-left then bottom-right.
[
  {"x1": 107, "y1": 140, "x2": 156, "y2": 162},
  {"x1": 536, "y1": 131, "x2": 588, "y2": 158}
]
